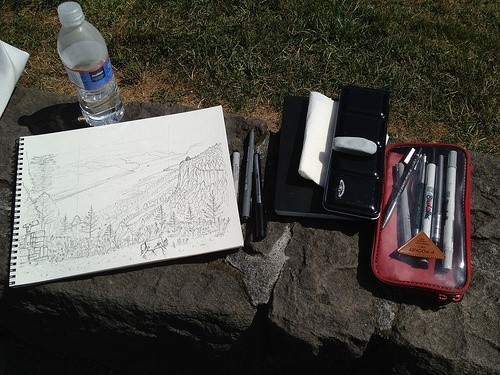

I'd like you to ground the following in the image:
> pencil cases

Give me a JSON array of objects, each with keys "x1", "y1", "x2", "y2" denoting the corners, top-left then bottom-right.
[{"x1": 371, "y1": 141, "x2": 472, "y2": 298}]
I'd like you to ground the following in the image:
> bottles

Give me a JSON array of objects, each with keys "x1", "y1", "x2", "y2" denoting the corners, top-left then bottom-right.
[{"x1": 54, "y1": 2, "x2": 125, "y2": 127}]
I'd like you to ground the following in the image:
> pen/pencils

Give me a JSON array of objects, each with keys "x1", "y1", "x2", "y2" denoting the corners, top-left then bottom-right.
[
  {"x1": 252, "y1": 153, "x2": 266, "y2": 241},
  {"x1": 242, "y1": 128, "x2": 255, "y2": 242},
  {"x1": 378, "y1": 139, "x2": 468, "y2": 275},
  {"x1": 231, "y1": 150, "x2": 242, "y2": 200}
]
